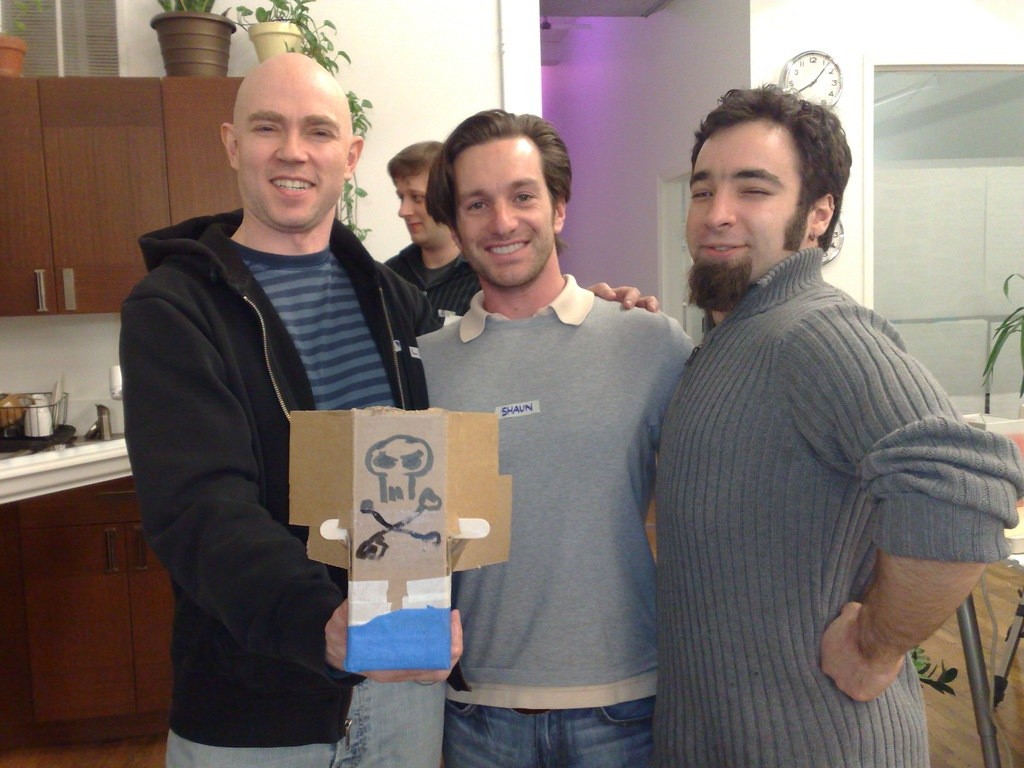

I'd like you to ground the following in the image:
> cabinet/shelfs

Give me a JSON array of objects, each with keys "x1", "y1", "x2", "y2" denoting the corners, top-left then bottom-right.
[
  {"x1": 0, "y1": 76, "x2": 244, "y2": 316},
  {"x1": 19, "y1": 475, "x2": 170, "y2": 744}
]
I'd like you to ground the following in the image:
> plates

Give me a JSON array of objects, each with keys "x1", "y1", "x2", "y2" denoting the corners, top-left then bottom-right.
[
  {"x1": 83, "y1": 421, "x2": 99, "y2": 439},
  {"x1": 51, "y1": 375, "x2": 61, "y2": 426}
]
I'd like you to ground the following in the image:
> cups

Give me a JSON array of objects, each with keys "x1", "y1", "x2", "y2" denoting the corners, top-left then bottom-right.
[
  {"x1": 109, "y1": 365, "x2": 123, "y2": 400},
  {"x1": 0, "y1": 392, "x2": 53, "y2": 436}
]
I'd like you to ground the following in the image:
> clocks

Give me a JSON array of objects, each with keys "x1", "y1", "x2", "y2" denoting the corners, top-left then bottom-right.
[{"x1": 780, "y1": 50, "x2": 843, "y2": 111}]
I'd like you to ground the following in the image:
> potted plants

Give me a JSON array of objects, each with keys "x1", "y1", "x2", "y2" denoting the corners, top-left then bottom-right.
[
  {"x1": 221, "y1": 0, "x2": 373, "y2": 242},
  {"x1": 150, "y1": 0, "x2": 237, "y2": 76},
  {"x1": 0, "y1": 0, "x2": 45, "y2": 76}
]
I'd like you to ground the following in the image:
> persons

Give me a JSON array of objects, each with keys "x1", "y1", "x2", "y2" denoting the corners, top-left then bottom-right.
[
  {"x1": 651, "y1": 82, "x2": 1024, "y2": 768},
  {"x1": 118, "y1": 51, "x2": 657, "y2": 768},
  {"x1": 380, "y1": 141, "x2": 482, "y2": 325},
  {"x1": 417, "y1": 109, "x2": 695, "y2": 768}
]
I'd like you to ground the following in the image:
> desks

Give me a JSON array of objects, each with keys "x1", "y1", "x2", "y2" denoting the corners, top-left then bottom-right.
[{"x1": 936, "y1": 392, "x2": 1024, "y2": 768}]
